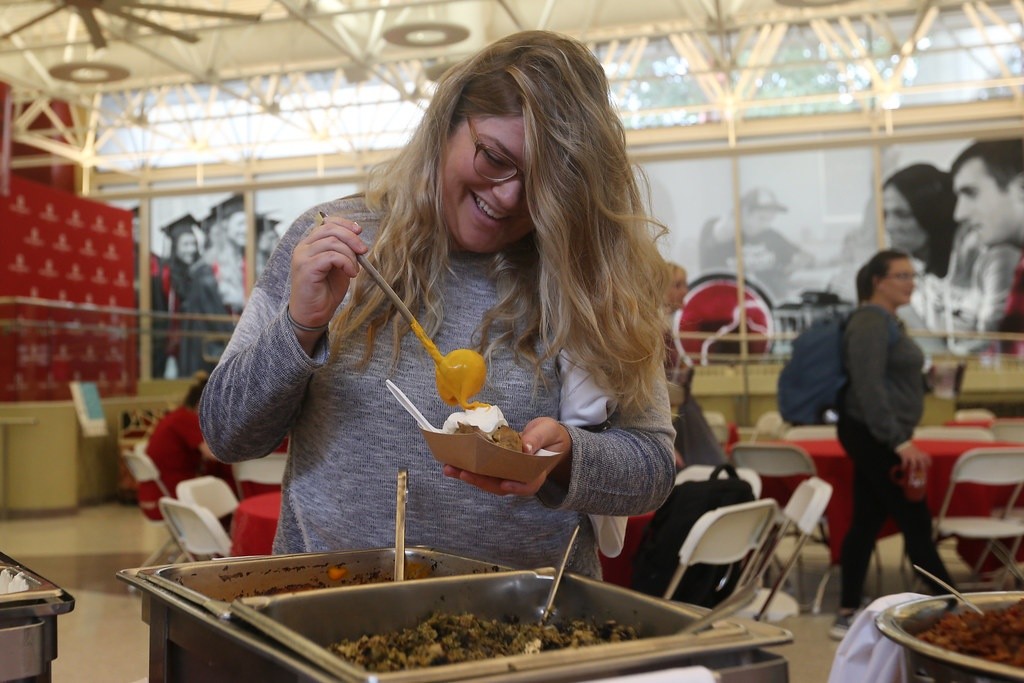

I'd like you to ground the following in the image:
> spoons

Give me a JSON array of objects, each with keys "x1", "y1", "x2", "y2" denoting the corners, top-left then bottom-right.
[{"x1": 320, "y1": 211, "x2": 486, "y2": 401}]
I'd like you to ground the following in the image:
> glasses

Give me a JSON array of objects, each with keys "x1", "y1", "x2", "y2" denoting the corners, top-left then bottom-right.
[
  {"x1": 883, "y1": 273, "x2": 919, "y2": 284},
  {"x1": 458, "y1": 108, "x2": 527, "y2": 183}
]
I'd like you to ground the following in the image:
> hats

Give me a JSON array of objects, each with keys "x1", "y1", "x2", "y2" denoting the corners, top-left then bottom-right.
[{"x1": 159, "y1": 194, "x2": 282, "y2": 241}]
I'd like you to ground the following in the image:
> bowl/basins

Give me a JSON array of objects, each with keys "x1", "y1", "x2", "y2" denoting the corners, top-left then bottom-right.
[{"x1": 875, "y1": 591, "x2": 1024, "y2": 683}]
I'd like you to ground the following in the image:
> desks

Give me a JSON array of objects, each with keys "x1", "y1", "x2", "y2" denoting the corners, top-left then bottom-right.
[
  {"x1": 760, "y1": 441, "x2": 1024, "y2": 614},
  {"x1": 230, "y1": 490, "x2": 655, "y2": 592}
]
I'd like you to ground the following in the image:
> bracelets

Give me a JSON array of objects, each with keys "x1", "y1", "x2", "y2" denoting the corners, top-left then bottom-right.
[{"x1": 287, "y1": 310, "x2": 329, "y2": 332}]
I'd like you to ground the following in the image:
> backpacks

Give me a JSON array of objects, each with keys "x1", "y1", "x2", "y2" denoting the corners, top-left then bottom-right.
[
  {"x1": 632, "y1": 462, "x2": 754, "y2": 608},
  {"x1": 776, "y1": 304, "x2": 901, "y2": 426}
]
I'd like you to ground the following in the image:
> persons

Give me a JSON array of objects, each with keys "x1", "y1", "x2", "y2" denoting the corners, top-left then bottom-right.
[
  {"x1": 197, "y1": 30, "x2": 677, "y2": 583},
  {"x1": 881, "y1": 138, "x2": 1024, "y2": 355},
  {"x1": 130, "y1": 195, "x2": 280, "y2": 379},
  {"x1": 145, "y1": 377, "x2": 238, "y2": 535},
  {"x1": 827, "y1": 249, "x2": 958, "y2": 641},
  {"x1": 654, "y1": 261, "x2": 731, "y2": 470}
]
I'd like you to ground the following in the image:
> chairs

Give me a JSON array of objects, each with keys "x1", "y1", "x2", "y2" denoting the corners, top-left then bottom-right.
[
  {"x1": 122, "y1": 439, "x2": 287, "y2": 593},
  {"x1": 663, "y1": 408, "x2": 1024, "y2": 623}
]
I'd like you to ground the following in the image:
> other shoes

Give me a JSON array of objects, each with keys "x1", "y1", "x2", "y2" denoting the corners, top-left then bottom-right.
[{"x1": 829, "y1": 613, "x2": 853, "y2": 637}]
